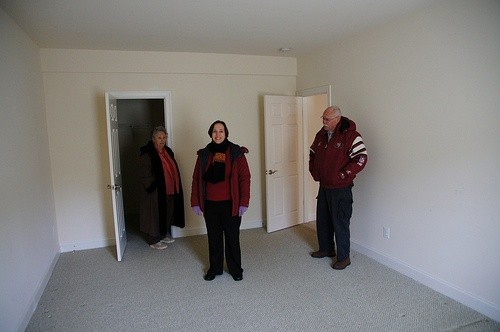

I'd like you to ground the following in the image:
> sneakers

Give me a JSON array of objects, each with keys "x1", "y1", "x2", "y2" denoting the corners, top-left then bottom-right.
[
  {"x1": 311, "y1": 250, "x2": 336, "y2": 258},
  {"x1": 332, "y1": 257, "x2": 351, "y2": 269},
  {"x1": 150, "y1": 242, "x2": 168, "y2": 249},
  {"x1": 160, "y1": 236, "x2": 175, "y2": 243}
]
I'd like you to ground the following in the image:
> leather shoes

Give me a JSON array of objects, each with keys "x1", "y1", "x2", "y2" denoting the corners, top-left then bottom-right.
[
  {"x1": 230, "y1": 271, "x2": 242, "y2": 280},
  {"x1": 205, "y1": 271, "x2": 223, "y2": 280}
]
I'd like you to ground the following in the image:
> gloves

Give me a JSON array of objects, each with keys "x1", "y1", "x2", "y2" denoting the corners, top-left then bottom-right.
[
  {"x1": 238, "y1": 206, "x2": 248, "y2": 216},
  {"x1": 192, "y1": 206, "x2": 203, "y2": 215}
]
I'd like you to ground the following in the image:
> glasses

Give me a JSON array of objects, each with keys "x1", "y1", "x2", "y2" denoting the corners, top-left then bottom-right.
[{"x1": 320, "y1": 116, "x2": 338, "y2": 122}]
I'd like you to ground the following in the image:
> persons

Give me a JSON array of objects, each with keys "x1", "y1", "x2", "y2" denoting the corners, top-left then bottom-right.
[
  {"x1": 138, "y1": 126, "x2": 186, "y2": 250},
  {"x1": 190, "y1": 120, "x2": 251, "y2": 281},
  {"x1": 308, "y1": 106, "x2": 368, "y2": 270}
]
